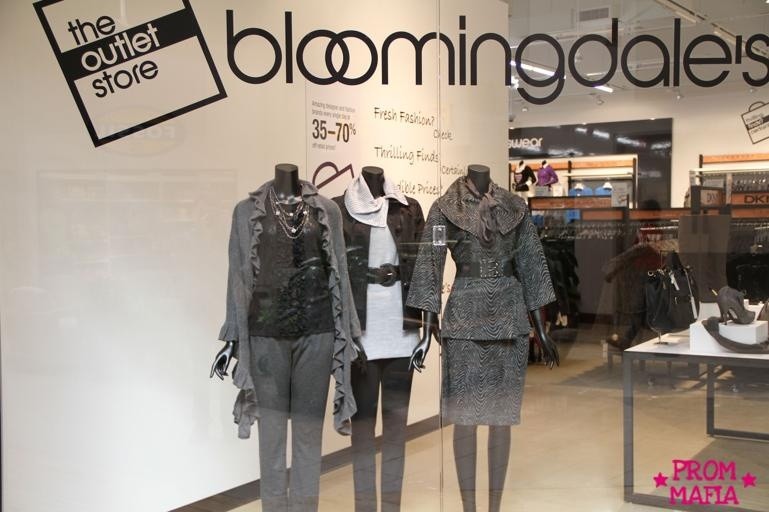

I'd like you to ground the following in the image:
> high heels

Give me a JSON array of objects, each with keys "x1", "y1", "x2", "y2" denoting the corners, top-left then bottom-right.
[{"x1": 718, "y1": 285, "x2": 756, "y2": 325}]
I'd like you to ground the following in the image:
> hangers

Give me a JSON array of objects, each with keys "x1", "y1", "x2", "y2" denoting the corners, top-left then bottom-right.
[
  {"x1": 531, "y1": 221, "x2": 683, "y2": 260},
  {"x1": 727, "y1": 173, "x2": 769, "y2": 193},
  {"x1": 727, "y1": 218, "x2": 769, "y2": 254}
]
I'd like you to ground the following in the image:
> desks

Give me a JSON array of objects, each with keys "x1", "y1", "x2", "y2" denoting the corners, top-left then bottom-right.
[{"x1": 620, "y1": 326, "x2": 768, "y2": 512}]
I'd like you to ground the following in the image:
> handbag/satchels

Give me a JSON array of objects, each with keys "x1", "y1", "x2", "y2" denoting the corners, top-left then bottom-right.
[{"x1": 645, "y1": 252, "x2": 701, "y2": 331}]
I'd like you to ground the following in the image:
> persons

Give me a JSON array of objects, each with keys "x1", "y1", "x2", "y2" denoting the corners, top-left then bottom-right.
[
  {"x1": 604, "y1": 198, "x2": 666, "y2": 351},
  {"x1": 403, "y1": 164, "x2": 562, "y2": 512},
  {"x1": 535, "y1": 159, "x2": 559, "y2": 190},
  {"x1": 208, "y1": 161, "x2": 360, "y2": 512},
  {"x1": 512, "y1": 158, "x2": 537, "y2": 191},
  {"x1": 332, "y1": 163, "x2": 440, "y2": 512}
]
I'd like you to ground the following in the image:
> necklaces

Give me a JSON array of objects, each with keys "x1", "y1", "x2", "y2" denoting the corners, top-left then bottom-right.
[{"x1": 267, "y1": 185, "x2": 315, "y2": 242}]
